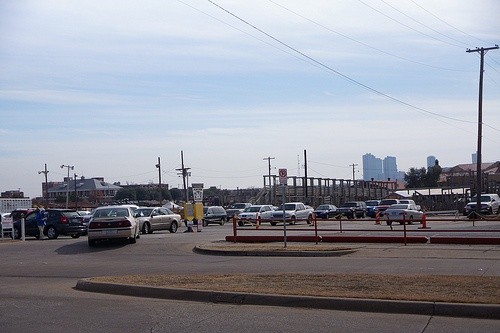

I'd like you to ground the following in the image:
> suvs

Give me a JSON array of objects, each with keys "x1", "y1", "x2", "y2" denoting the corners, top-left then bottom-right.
[
  {"x1": 237, "y1": 205, "x2": 274, "y2": 226},
  {"x1": 336, "y1": 201, "x2": 367, "y2": 220},
  {"x1": 192, "y1": 205, "x2": 227, "y2": 227},
  {"x1": 225, "y1": 203, "x2": 252, "y2": 222},
  {"x1": 365, "y1": 198, "x2": 421, "y2": 218},
  {"x1": 463, "y1": 194, "x2": 500, "y2": 216}
]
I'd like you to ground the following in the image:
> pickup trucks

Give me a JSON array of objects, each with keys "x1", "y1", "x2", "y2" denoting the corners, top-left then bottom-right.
[{"x1": 269, "y1": 202, "x2": 314, "y2": 226}]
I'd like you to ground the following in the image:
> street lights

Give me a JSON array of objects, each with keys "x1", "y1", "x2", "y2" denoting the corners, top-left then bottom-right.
[
  {"x1": 60, "y1": 164, "x2": 74, "y2": 209},
  {"x1": 155, "y1": 157, "x2": 162, "y2": 207},
  {"x1": 186, "y1": 172, "x2": 191, "y2": 203},
  {"x1": 37, "y1": 163, "x2": 49, "y2": 207}
]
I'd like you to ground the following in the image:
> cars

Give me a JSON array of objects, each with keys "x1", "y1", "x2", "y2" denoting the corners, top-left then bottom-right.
[
  {"x1": 0, "y1": 207, "x2": 93, "y2": 240},
  {"x1": 87, "y1": 206, "x2": 141, "y2": 247},
  {"x1": 312, "y1": 205, "x2": 338, "y2": 220},
  {"x1": 133, "y1": 207, "x2": 182, "y2": 234},
  {"x1": 382, "y1": 204, "x2": 424, "y2": 225},
  {"x1": 108, "y1": 204, "x2": 144, "y2": 217}
]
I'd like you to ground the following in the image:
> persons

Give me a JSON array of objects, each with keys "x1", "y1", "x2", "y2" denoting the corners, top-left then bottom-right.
[{"x1": 37, "y1": 208, "x2": 47, "y2": 239}]
